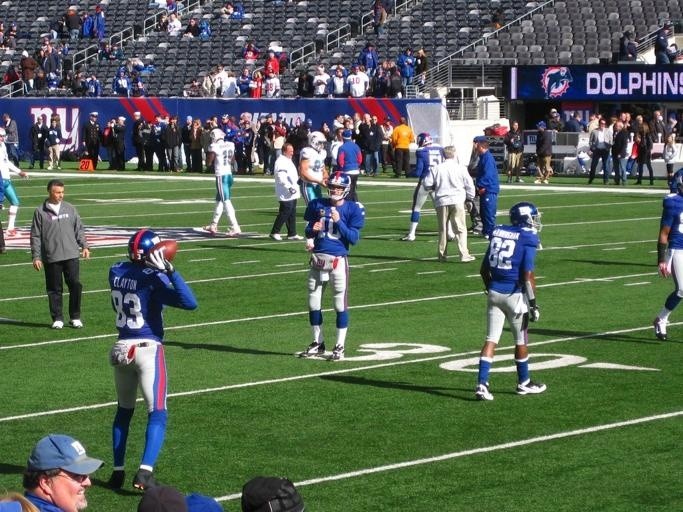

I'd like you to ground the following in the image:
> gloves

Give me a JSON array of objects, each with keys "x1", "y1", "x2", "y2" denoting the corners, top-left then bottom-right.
[
  {"x1": 144, "y1": 248, "x2": 175, "y2": 275},
  {"x1": 529, "y1": 304, "x2": 540, "y2": 322}
]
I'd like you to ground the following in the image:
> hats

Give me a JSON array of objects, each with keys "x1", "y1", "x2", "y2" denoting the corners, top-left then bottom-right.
[
  {"x1": 550, "y1": 108, "x2": 558, "y2": 113},
  {"x1": 535, "y1": 121, "x2": 546, "y2": 129},
  {"x1": 342, "y1": 129, "x2": 352, "y2": 140},
  {"x1": 222, "y1": 114, "x2": 229, "y2": 120},
  {"x1": 89, "y1": 111, "x2": 99, "y2": 118},
  {"x1": 237, "y1": 113, "x2": 314, "y2": 129},
  {"x1": 118, "y1": 117, "x2": 126, "y2": 121},
  {"x1": 27, "y1": 432, "x2": 106, "y2": 476},
  {"x1": 383, "y1": 116, "x2": 394, "y2": 122},
  {"x1": 133, "y1": 111, "x2": 140, "y2": 116}
]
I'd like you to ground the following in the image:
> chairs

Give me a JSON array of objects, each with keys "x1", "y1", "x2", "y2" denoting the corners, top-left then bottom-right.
[{"x1": 1, "y1": 0, "x2": 683, "y2": 101}]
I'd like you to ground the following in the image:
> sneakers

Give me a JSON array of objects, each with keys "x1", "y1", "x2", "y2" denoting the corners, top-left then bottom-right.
[
  {"x1": 400, "y1": 233, "x2": 416, "y2": 241},
  {"x1": 652, "y1": 316, "x2": 669, "y2": 341},
  {"x1": 51, "y1": 320, "x2": 65, "y2": 328},
  {"x1": 201, "y1": 222, "x2": 218, "y2": 234},
  {"x1": 67, "y1": 317, "x2": 84, "y2": 329},
  {"x1": 287, "y1": 234, "x2": 305, "y2": 240},
  {"x1": 269, "y1": 232, "x2": 283, "y2": 241},
  {"x1": 447, "y1": 234, "x2": 458, "y2": 242},
  {"x1": 28, "y1": 164, "x2": 63, "y2": 170},
  {"x1": 293, "y1": 339, "x2": 326, "y2": 358},
  {"x1": 506, "y1": 177, "x2": 655, "y2": 185},
  {"x1": 515, "y1": 377, "x2": 547, "y2": 395},
  {"x1": 467, "y1": 226, "x2": 490, "y2": 240},
  {"x1": 131, "y1": 468, "x2": 162, "y2": 491},
  {"x1": 327, "y1": 344, "x2": 345, "y2": 362},
  {"x1": 459, "y1": 253, "x2": 476, "y2": 263},
  {"x1": 474, "y1": 382, "x2": 495, "y2": 401},
  {"x1": 225, "y1": 226, "x2": 242, "y2": 236},
  {"x1": 5, "y1": 228, "x2": 22, "y2": 238},
  {"x1": 106, "y1": 469, "x2": 125, "y2": 490}
]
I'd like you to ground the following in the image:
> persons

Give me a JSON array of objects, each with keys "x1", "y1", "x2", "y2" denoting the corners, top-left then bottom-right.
[
  {"x1": 107, "y1": 228, "x2": 198, "y2": 491},
  {"x1": 475, "y1": 201, "x2": 549, "y2": 401},
  {"x1": 0, "y1": 430, "x2": 104, "y2": 510}
]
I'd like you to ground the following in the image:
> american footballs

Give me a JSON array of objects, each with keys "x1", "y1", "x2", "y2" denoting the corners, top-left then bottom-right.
[{"x1": 146, "y1": 240, "x2": 177, "y2": 264}]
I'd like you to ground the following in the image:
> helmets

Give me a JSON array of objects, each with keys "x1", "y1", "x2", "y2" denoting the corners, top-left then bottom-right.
[
  {"x1": 0, "y1": 127, "x2": 6, "y2": 137},
  {"x1": 509, "y1": 202, "x2": 537, "y2": 227},
  {"x1": 326, "y1": 171, "x2": 353, "y2": 201},
  {"x1": 127, "y1": 228, "x2": 160, "y2": 262},
  {"x1": 669, "y1": 167, "x2": 683, "y2": 196},
  {"x1": 209, "y1": 128, "x2": 225, "y2": 143},
  {"x1": 308, "y1": 131, "x2": 327, "y2": 153},
  {"x1": 416, "y1": 132, "x2": 432, "y2": 148}
]
